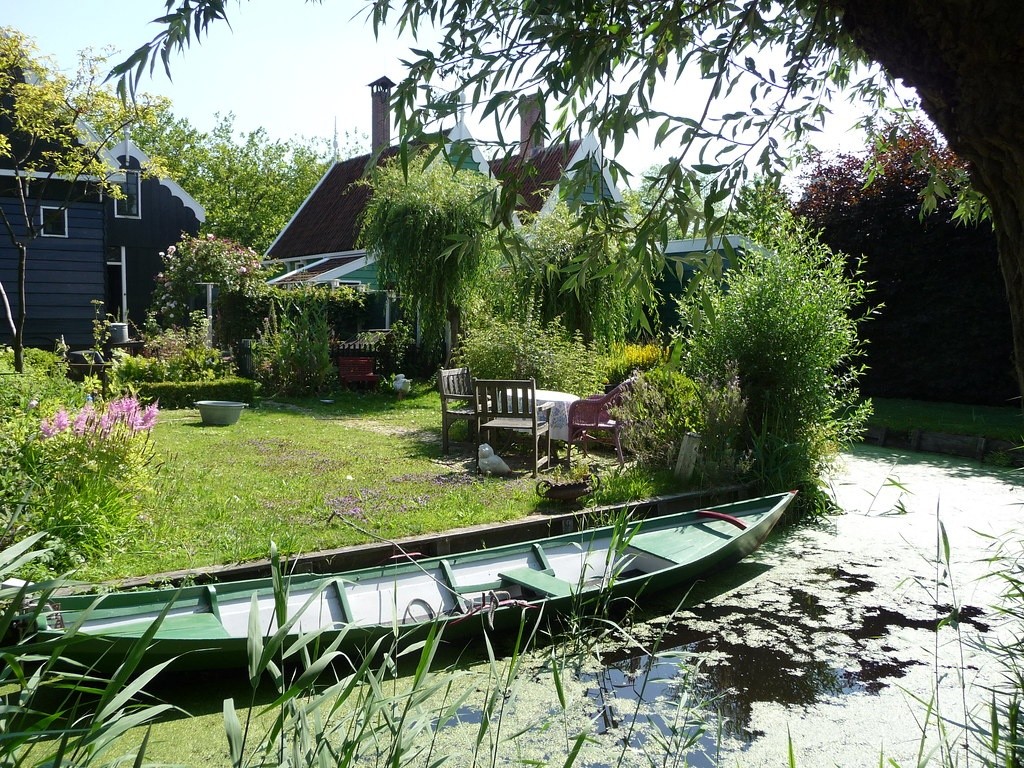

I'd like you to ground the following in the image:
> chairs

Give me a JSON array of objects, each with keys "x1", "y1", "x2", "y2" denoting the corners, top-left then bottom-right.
[
  {"x1": 567, "y1": 376, "x2": 640, "y2": 468},
  {"x1": 437, "y1": 366, "x2": 554, "y2": 479}
]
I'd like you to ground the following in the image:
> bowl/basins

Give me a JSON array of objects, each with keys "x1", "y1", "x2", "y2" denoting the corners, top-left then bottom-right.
[{"x1": 196, "y1": 400, "x2": 249, "y2": 426}]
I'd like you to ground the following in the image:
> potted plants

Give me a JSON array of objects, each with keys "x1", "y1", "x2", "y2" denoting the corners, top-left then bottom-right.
[{"x1": 106, "y1": 304, "x2": 130, "y2": 341}]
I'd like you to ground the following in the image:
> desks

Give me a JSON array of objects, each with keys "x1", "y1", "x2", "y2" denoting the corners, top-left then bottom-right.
[{"x1": 497, "y1": 386, "x2": 581, "y2": 461}]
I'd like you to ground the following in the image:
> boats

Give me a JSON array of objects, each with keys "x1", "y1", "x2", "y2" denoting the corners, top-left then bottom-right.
[{"x1": 32, "y1": 489, "x2": 798, "y2": 674}]
